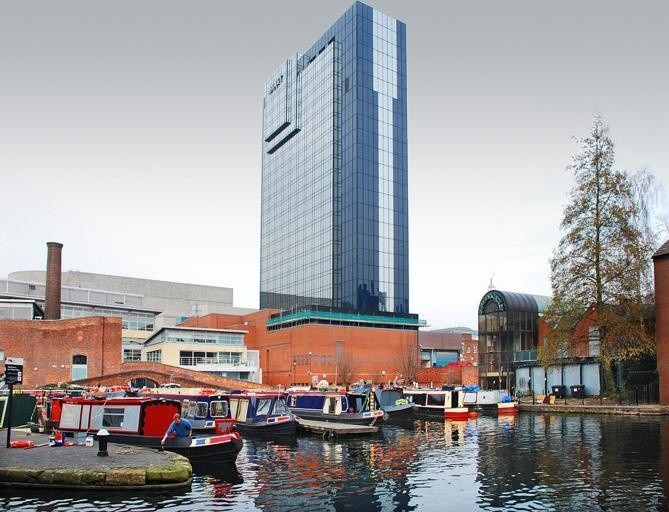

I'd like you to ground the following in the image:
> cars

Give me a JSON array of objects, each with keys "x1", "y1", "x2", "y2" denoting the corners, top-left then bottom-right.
[
  {"x1": 66, "y1": 384, "x2": 80, "y2": 390},
  {"x1": 160, "y1": 382, "x2": 182, "y2": 389}
]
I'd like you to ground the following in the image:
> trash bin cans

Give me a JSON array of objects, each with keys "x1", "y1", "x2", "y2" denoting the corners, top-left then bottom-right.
[
  {"x1": 570, "y1": 385, "x2": 585, "y2": 397},
  {"x1": 551, "y1": 385, "x2": 566, "y2": 398}
]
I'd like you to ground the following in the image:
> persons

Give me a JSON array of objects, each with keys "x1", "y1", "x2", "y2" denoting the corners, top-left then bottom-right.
[{"x1": 160, "y1": 413, "x2": 192, "y2": 445}]
